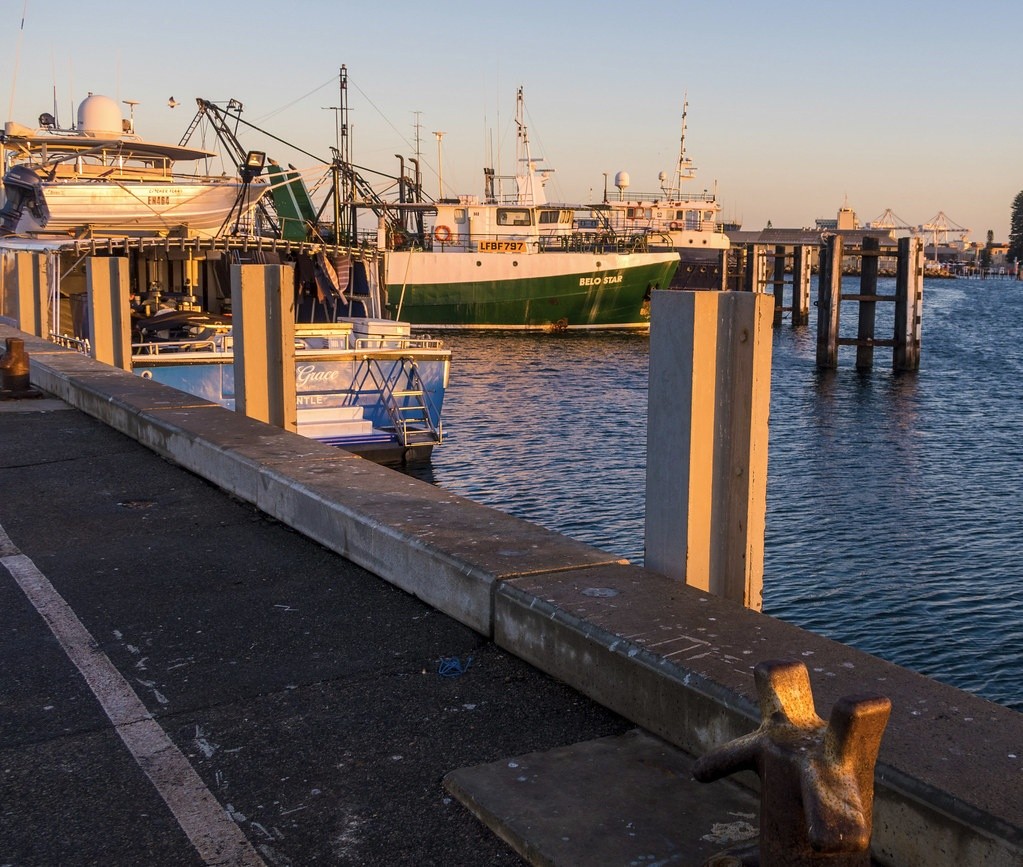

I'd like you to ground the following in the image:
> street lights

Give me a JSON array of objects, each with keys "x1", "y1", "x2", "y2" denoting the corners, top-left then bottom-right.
[{"x1": 432, "y1": 132, "x2": 447, "y2": 200}]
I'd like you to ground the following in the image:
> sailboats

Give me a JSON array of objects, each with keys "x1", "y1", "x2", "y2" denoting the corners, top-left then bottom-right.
[
  {"x1": 427, "y1": 85, "x2": 731, "y2": 292},
  {"x1": 305, "y1": 64, "x2": 682, "y2": 329}
]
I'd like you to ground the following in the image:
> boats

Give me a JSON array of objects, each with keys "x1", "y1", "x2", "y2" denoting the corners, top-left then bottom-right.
[{"x1": 1, "y1": 84, "x2": 452, "y2": 464}]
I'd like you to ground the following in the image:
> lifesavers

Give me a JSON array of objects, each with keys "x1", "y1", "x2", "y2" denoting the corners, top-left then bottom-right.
[
  {"x1": 669, "y1": 221, "x2": 678, "y2": 231},
  {"x1": 434, "y1": 223, "x2": 453, "y2": 242}
]
